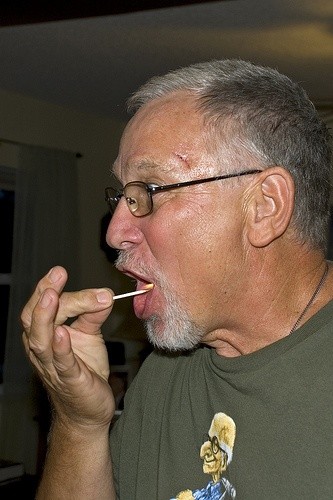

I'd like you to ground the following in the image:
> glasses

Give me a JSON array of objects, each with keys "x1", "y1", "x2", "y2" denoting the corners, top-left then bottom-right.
[{"x1": 100, "y1": 168, "x2": 264, "y2": 219}]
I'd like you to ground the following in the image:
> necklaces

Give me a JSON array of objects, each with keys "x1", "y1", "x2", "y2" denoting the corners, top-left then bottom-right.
[{"x1": 288, "y1": 261, "x2": 328, "y2": 336}]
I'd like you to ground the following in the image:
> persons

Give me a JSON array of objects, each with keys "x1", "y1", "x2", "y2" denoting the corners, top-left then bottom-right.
[{"x1": 20, "y1": 60, "x2": 333, "y2": 498}]
